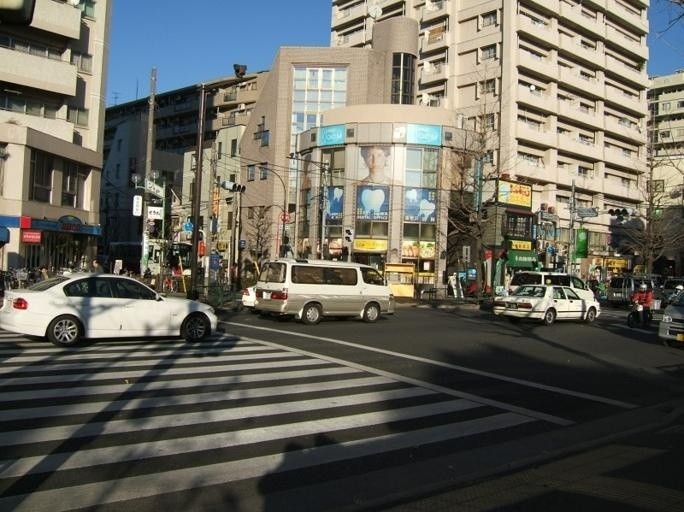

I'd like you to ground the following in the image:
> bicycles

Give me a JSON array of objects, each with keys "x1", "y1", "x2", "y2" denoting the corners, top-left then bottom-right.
[{"x1": 627, "y1": 302, "x2": 653, "y2": 328}]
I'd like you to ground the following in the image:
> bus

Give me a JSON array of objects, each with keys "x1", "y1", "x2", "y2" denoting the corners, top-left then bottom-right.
[{"x1": 105, "y1": 239, "x2": 204, "y2": 278}]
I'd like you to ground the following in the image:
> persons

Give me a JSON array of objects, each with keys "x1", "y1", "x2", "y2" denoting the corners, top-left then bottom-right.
[
  {"x1": 91, "y1": 259, "x2": 104, "y2": 272},
  {"x1": 357, "y1": 145, "x2": 393, "y2": 186},
  {"x1": 630, "y1": 283, "x2": 653, "y2": 328},
  {"x1": 447, "y1": 271, "x2": 458, "y2": 298},
  {"x1": 36, "y1": 265, "x2": 49, "y2": 283},
  {"x1": 118, "y1": 268, "x2": 132, "y2": 277},
  {"x1": 668, "y1": 284, "x2": 683, "y2": 304},
  {"x1": 302, "y1": 154, "x2": 311, "y2": 189}
]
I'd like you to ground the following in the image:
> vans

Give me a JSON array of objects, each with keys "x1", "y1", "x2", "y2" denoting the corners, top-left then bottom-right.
[
  {"x1": 254, "y1": 258, "x2": 395, "y2": 325},
  {"x1": 506, "y1": 271, "x2": 684, "y2": 319}
]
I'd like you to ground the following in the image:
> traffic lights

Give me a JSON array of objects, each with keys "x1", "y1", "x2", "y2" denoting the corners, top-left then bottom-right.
[
  {"x1": 283, "y1": 236, "x2": 289, "y2": 244},
  {"x1": 608, "y1": 209, "x2": 632, "y2": 217},
  {"x1": 219, "y1": 180, "x2": 246, "y2": 193}
]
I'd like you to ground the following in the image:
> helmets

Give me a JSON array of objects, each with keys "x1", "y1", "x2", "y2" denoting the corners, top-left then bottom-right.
[{"x1": 640, "y1": 284, "x2": 648, "y2": 292}]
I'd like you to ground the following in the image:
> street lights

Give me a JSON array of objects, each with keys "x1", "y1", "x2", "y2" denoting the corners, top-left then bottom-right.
[
  {"x1": 256, "y1": 164, "x2": 287, "y2": 257},
  {"x1": 131, "y1": 169, "x2": 166, "y2": 292}
]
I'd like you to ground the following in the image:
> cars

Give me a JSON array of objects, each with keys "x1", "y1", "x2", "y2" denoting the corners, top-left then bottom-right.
[
  {"x1": 492, "y1": 284, "x2": 597, "y2": 325},
  {"x1": 0, "y1": 273, "x2": 256, "y2": 347},
  {"x1": 55, "y1": 267, "x2": 76, "y2": 276},
  {"x1": 658, "y1": 289, "x2": 684, "y2": 347}
]
